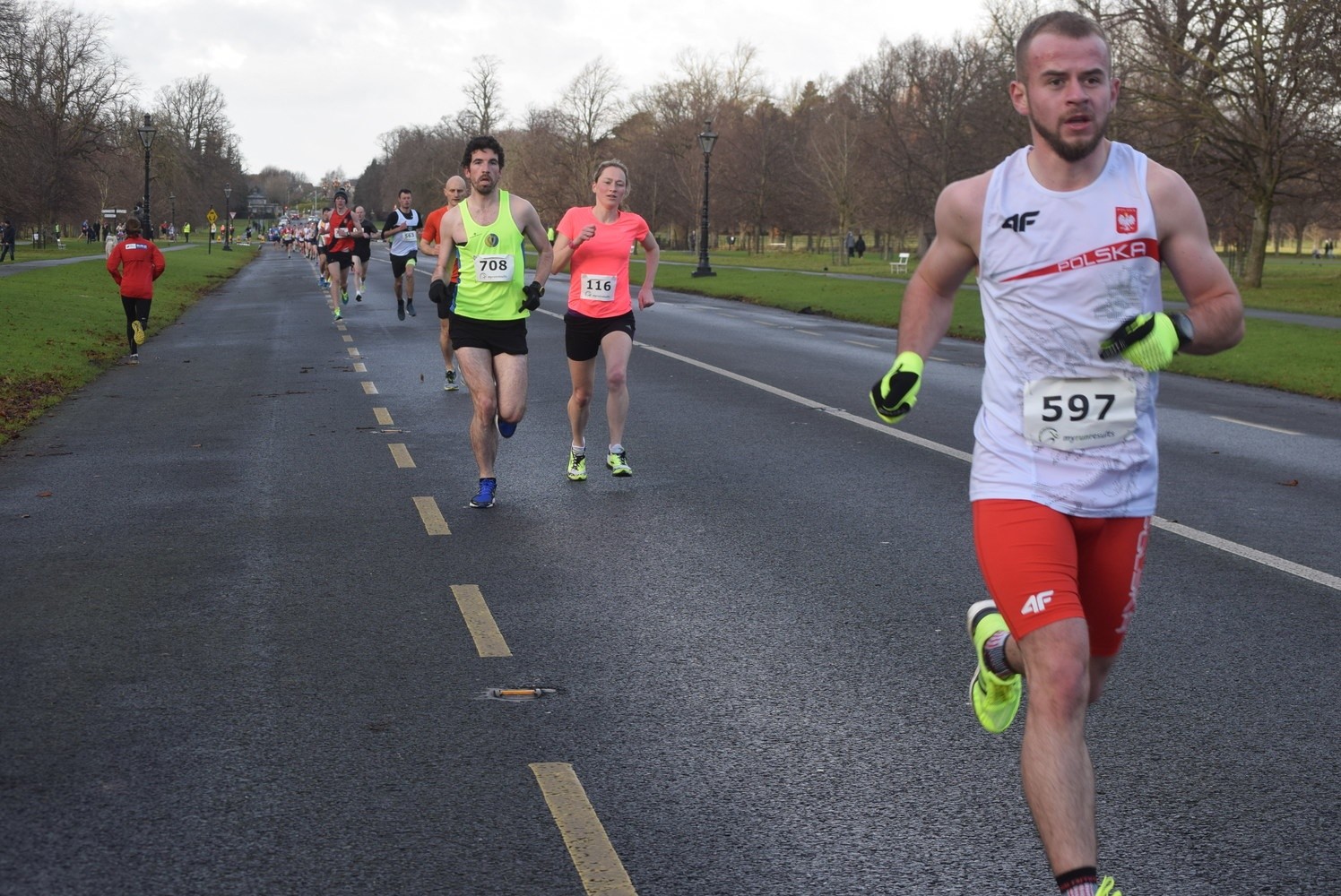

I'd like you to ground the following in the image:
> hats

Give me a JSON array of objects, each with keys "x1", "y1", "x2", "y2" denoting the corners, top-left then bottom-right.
[{"x1": 334, "y1": 187, "x2": 348, "y2": 204}]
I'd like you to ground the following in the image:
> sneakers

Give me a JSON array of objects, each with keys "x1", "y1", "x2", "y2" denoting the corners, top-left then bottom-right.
[
  {"x1": 495, "y1": 415, "x2": 517, "y2": 437},
  {"x1": 966, "y1": 598, "x2": 1022, "y2": 733},
  {"x1": 606, "y1": 445, "x2": 632, "y2": 476},
  {"x1": 470, "y1": 477, "x2": 497, "y2": 507},
  {"x1": 457, "y1": 366, "x2": 467, "y2": 386},
  {"x1": 124, "y1": 354, "x2": 139, "y2": 365},
  {"x1": 360, "y1": 284, "x2": 366, "y2": 293},
  {"x1": 444, "y1": 365, "x2": 460, "y2": 390},
  {"x1": 406, "y1": 301, "x2": 416, "y2": 316},
  {"x1": 334, "y1": 307, "x2": 343, "y2": 320},
  {"x1": 131, "y1": 321, "x2": 144, "y2": 345},
  {"x1": 341, "y1": 288, "x2": 349, "y2": 304},
  {"x1": 318, "y1": 276, "x2": 331, "y2": 290},
  {"x1": 398, "y1": 299, "x2": 405, "y2": 320},
  {"x1": 356, "y1": 292, "x2": 361, "y2": 302},
  {"x1": 567, "y1": 449, "x2": 588, "y2": 481}
]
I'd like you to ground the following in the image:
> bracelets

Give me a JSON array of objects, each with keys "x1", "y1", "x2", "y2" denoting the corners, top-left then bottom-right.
[{"x1": 568, "y1": 239, "x2": 580, "y2": 250}]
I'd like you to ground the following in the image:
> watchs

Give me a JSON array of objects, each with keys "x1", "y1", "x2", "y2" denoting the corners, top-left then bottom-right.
[
  {"x1": 532, "y1": 281, "x2": 546, "y2": 298},
  {"x1": 1162, "y1": 308, "x2": 1195, "y2": 358},
  {"x1": 347, "y1": 232, "x2": 350, "y2": 237}
]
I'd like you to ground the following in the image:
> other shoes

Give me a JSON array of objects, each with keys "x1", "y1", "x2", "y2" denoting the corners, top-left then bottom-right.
[
  {"x1": 305, "y1": 254, "x2": 319, "y2": 264},
  {"x1": 289, "y1": 255, "x2": 291, "y2": 258}
]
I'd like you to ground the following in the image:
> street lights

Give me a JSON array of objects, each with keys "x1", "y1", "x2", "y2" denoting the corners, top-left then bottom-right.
[
  {"x1": 136, "y1": 112, "x2": 158, "y2": 241},
  {"x1": 222, "y1": 181, "x2": 232, "y2": 251},
  {"x1": 169, "y1": 192, "x2": 176, "y2": 228},
  {"x1": 690, "y1": 120, "x2": 721, "y2": 277}
]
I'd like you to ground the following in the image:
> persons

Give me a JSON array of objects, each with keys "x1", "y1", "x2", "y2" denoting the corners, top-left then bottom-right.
[
  {"x1": 551, "y1": 158, "x2": 660, "y2": 481},
  {"x1": 690, "y1": 230, "x2": 867, "y2": 260},
  {"x1": 106, "y1": 219, "x2": 166, "y2": 364},
  {"x1": 871, "y1": 13, "x2": 1247, "y2": 896},
  {"x1": 381, "y1": 189, "x2": 423, "y2": 322},
  {"x1": 420, "y1": 175, "x2": 469, "y2": 391},
  {"x1": 0, "y1": 206, "x2": 394, "y2": 290},
  {"x1": 429, "y1": 135, "x2": 554, "y2": 509},
  {"x1": 351, "y1": 205, "x2": 380, "y2": 303},
  {"x1": 318, "y1": 187, "x2": 364, "y2": 321}
]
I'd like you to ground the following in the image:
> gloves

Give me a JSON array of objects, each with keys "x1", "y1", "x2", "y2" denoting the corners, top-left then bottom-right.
[
  {"x1": 869, "y1": 350, "x2": 924, "y2": 421},
  {"x1": 1098, "y1": 309, "x2": 1194, "y2": 372},
  {"x1": 518, "y1": 281, "x2": 542, "y2": 313},
  {"x1": 429, "y1": 279, "x2": 446, "y2": 303}
]
